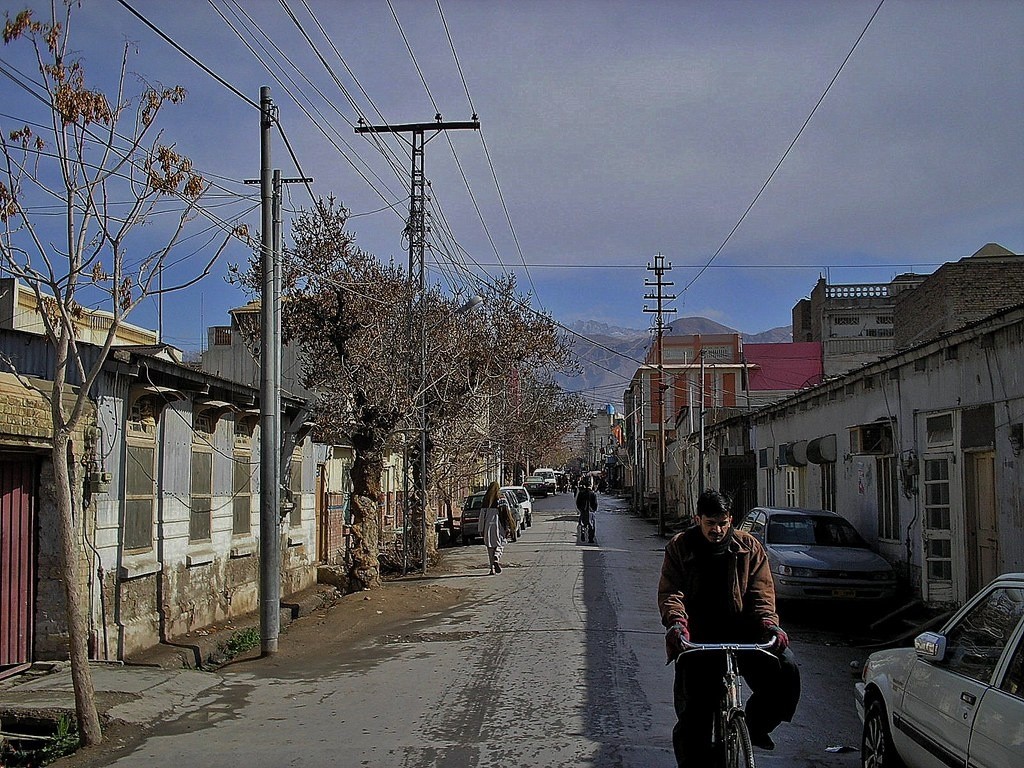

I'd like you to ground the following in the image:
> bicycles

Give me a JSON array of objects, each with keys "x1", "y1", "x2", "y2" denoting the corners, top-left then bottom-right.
[{"x1": 675, "y1": 631, "x2": 782, "y2": 768}]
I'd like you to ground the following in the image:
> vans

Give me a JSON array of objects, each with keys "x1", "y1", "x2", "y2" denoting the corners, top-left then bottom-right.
[{"x1": 533, "y1": 468, "x2": 558, "y2": 495}]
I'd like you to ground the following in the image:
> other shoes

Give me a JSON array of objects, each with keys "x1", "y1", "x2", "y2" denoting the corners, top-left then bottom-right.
[
  {"x1": 490, "y1": 571, "x2": 495, "y2": 575},
  {"x1": 581, "y1": 532, "x2": 586, "y2": 542},
  {"x1": 588, "y1": 539, "x2": 595, "y2": 544},
  {"x1": 493, "y1": 561, "x2": 501, "y2": 573},
  {"x1": 749, "y1": 731, "x2": 774, "y2": 750}
]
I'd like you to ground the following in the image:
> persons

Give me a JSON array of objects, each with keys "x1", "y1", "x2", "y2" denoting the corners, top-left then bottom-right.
[
  {"x1": 577, "y1": 481, "x2": 598, "y2": 543},
  {"x1": 478, "y1": 482, "x2": 516, "y2": 575},
  {"x1": 658, "y1": 487, "x2": 801, "y2": 768},
  {"x1": 558, "y1": 473, "x2": 591, "y2": 498}
]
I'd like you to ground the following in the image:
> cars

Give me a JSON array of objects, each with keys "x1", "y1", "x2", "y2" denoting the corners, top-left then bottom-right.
[
  {"x1": 435, "y1": 516, "x2": 461, "y2": 549},
  {"x1": 554, "y1": 470, "x2": 577, "y2": 492},
  {"x1": 500, "y1": 486, "x2": 534, "y2": 530},
  {"x1": 522, "y1": 476, "x2": 550, "y2": 499},
  {"x1": 852, "y1": 573, "x2": 1024, "y2": 768},
  {"x1": 463, "y1": 491, "x2": 523, "y2": 542},
  {"x1": 734, "y1": 506, "x2": 898, "y2": 629}
]
